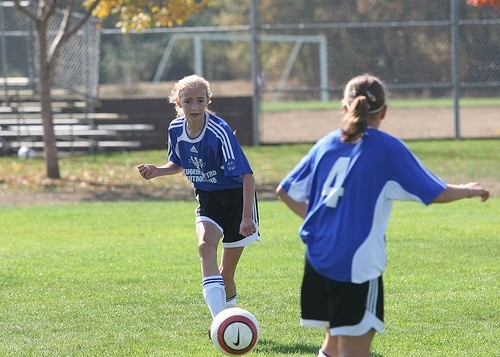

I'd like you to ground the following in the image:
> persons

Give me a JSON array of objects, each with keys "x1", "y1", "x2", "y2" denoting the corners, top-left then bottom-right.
[
  {"x1": 137, "y1": 75, "x2": 263, "y2": 340},
  {"x1": 274, "y1": 72, "x2": 490, "y2": 357}
]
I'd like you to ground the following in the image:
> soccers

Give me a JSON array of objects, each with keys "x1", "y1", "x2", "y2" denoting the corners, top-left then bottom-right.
[{"x1": 211, "y1": 307, "x2": 260, "y2": 357}]
